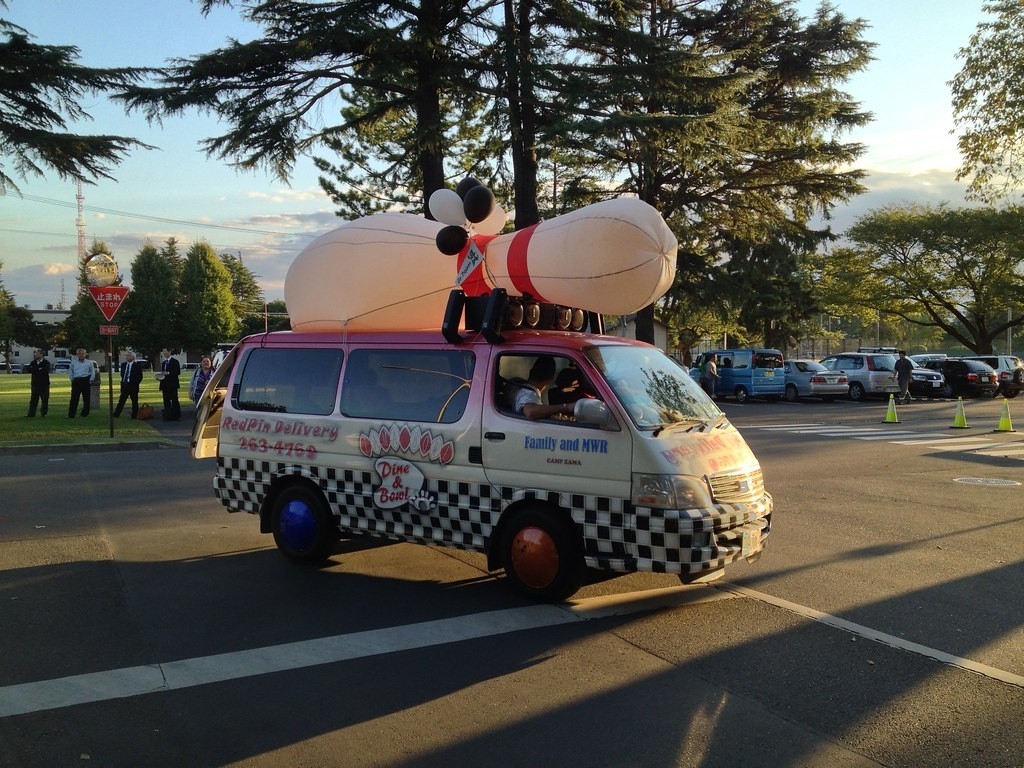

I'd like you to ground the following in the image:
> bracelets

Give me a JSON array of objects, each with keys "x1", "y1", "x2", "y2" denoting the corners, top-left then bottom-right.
[{"x1": 562, "y1": 404, "x2": 567, "y2": 412}]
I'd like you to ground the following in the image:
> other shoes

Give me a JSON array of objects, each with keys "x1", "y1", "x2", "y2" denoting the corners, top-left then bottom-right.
[
  {"x1": 907, "y1": 399, "x2": 910, "y2": 403},
  {"x1": 900, "y1": 402, "x2": 903, "y2": 405},
  {"x1": 25, "y1": 415, "x2": 30, "y2": 417},
  {"x1": 179, "y1": 418, "x2": 182, "y2": 421},
  {"x1": 43, "y1": 415, "x2": 46, "y2": 417}
]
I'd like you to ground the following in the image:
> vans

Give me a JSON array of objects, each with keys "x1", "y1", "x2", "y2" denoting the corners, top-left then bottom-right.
[
  {"x1": 88, "y1": 360, "x2": 99, "y2": 372},
  {"x1": 910, "y1": 354, "x2": 947, "y2": 360},
  {"x1": 688, "y1": 348, "x2": 785, "y2": 403},
  {"x1": 817, "y1": 352, "x2": 904, "y2": 400},
  {"x1": 134, "y1": 359, "x2": 153, "y2": 372},
  {"x1": 180, "y1": 363, "x2": 201, "y2": 370},
  {"x1": 190, "y1": 286, "x2": 774, "y2": 604}
]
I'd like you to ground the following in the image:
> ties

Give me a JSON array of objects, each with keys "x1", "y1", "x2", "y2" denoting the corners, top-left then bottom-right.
[
  {"x1": 166, "y1": 360, "x2": 168, "y2": 368},
  {"x1": 125, "y1": 364, "x2": 129, "y2": 383}
]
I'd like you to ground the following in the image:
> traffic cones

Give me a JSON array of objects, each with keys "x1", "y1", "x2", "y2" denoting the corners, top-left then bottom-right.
[
  {"x1": 949, "y1": 396, "x2": 972, "y2": 429},
  {"x1": 993, "y1": 398, "x2": 1017, "y2": 431},
  {"x1": 881, "y1": 394, "x2": 902, "y2": 424}
]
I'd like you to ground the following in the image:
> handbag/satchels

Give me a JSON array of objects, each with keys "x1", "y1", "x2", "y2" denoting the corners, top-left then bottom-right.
[
  {"x1": 161, "y1": 405, "x2": 180, "y2": 421},
  {"x1": 137, "y1": 403, "x2": 154, "y2": 419}
]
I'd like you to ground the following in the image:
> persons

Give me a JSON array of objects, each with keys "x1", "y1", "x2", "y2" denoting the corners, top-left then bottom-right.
[
  {"x1": 113, "y1": 351, "x2": 143, "y2": 418},
  {"x1": 684, "y1": 351, "x2": 691, "y2": 366},
  {"x1": 705, "y1": 354, "x2": 719, "y2": 398},
  {"x1": 188, "y1": 357, "x2": 216, "y2": 415},
  {"x1": 512, "y1": 357, "x2": 601, "y2": 420},
  {"x1": 64, "y1": 349, "x2": 95, "y2": 418},
  {"x1": 24, "y1": 350, "x2": 51, "y2": 418},
  {"x1": 155, "y1": 347, "x2": 182, "y2": 421},
  {"x1": 893, "y1": 351, "x2": 914, "y2": 405}
]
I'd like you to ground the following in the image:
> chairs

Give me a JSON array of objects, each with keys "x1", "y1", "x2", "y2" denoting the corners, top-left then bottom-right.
[
  {"x1": 549, "y1": 367, "x2": 581, "y2": 404},
  {"x1": 502, "y1": 377, "x2": 527, "y2": 412}
]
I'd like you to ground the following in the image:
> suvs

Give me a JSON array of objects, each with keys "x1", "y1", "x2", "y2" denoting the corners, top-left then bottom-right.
[
  {"x1": 953, "y1": 355, "x2": 1024, "y2": 398},
  {"x1": 850, "y1": 346, "x2": 945, "y2": 395}
]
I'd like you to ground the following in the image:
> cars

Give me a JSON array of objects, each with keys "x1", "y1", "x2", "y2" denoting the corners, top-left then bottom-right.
[
  {"x1": 752, "y1": 359, "x2": 849, "y2": 402},
  {"x1": 915, "y1": 359, "x2": 1000, "y2": 400},
  {"x1": 0, "y1": 360, "x2": 71, "y2": 374}
]
